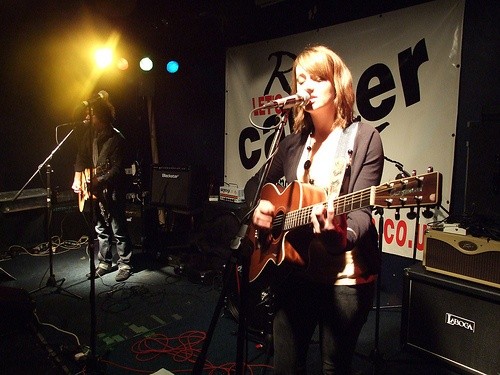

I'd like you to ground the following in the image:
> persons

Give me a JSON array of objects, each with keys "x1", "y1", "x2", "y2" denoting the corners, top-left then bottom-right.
[
  {"x1": 243, "y1": 44, "x2": 385, "y2": 375},
  {"x1": 71, "y1": 98, "x2": 134, "y2": 281}
]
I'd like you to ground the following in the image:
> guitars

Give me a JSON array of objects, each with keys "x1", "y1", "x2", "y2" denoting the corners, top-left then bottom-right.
[
  {"x1": 246, "y1": 166, "x2": 443, "y2": 284},
  {"x1": 78, "y1": 157, "x2": 113, "y2": 214}
]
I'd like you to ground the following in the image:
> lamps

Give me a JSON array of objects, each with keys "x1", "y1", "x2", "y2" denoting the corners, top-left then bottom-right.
[{"x1": 138, "y1": 56, "x2": 154, "y2": 74}]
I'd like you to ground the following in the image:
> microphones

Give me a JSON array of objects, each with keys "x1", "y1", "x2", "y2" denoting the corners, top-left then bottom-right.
[
  {"x1": 76, "y1": 89, "x2": 108, "y2": 112},
  {"x1": 262, "y1": 90, "x2": 310, "y2": 109},
  {"x1": 64, "y1": 120, "x2": 89, "y2": 129}
]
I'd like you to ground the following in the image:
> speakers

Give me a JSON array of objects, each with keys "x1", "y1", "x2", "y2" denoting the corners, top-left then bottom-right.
[
  {"x1": 397, "y1": 266, "x2": 500, "y2": 375},
  {"x1": 150, "y1": 167, "x2": 200, "y2": 208}
]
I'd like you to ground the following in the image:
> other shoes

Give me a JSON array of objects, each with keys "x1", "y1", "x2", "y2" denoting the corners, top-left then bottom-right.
[
  {"x1": 115, "y1": 268, "x2": 130, "y2": 281},
  {"x1": 86, "y1": 266, "x2": 113, "y2": 279}
]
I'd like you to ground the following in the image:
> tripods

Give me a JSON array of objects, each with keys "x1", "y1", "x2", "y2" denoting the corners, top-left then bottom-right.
[{"x1": 8, "y1": 123, "x2": 83, "y2": 302}]
